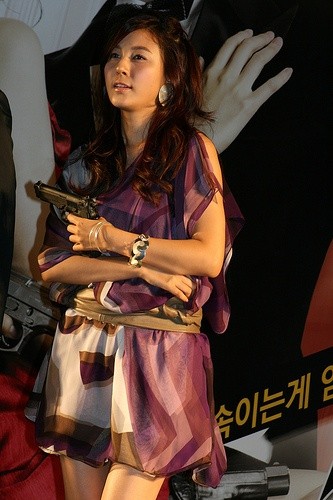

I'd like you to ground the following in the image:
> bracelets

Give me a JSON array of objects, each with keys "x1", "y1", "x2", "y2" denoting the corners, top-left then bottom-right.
[
  {"x1": 93, "y1": 222, "x2": 116, "y2": 255},
  {"x1": 87, "y1": 220, "x2": 109, "y2": 251},
  {"x1": 126, "y1": 232, "x2": 151, "y2": 269}
]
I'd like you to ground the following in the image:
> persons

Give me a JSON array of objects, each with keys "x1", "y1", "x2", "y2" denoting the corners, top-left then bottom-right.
[
  {"x1": 1, "y1": 0, "x2": 294, "y2": 290},
  {"x1": 0, "y1": 90, "x2": 17, "y2": 333},
  {"x1": 25, "y1": 8, "x2": 245, "y2": 500}
]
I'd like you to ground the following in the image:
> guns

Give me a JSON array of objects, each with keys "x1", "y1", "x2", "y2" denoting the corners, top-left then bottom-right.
[
  {"x1": 33, "y1": 181, "x2": 101, "y2": 260},
  {"x1": 1, "y1": 271, "x2": 59, "y2": 355}
]
